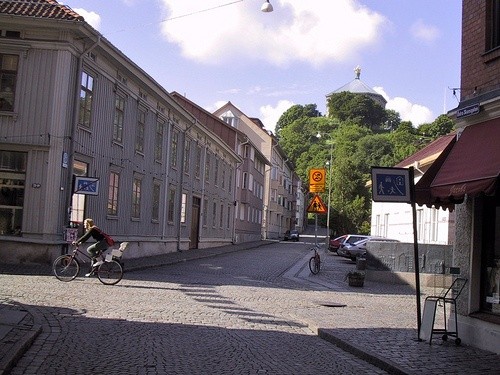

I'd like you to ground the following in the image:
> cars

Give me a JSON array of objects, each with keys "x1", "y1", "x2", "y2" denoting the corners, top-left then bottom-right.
[
  {"x1": 329, "y1": 235, "x2": 348, "y2": 252},
  {"x1": 284, "y1": 229, "x2": 299, "y2": 242},
  {"x1": 343, "y1": 237, "x2": 401, "y2": 264}
]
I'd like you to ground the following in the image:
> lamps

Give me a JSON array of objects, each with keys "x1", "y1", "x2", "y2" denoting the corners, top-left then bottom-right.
[
  {"x1": 261, "y1": 0, "x2": 274, "y2": 12},
  {"x1": 354, "y1": 65, "x2": 363, "y2": 80}
]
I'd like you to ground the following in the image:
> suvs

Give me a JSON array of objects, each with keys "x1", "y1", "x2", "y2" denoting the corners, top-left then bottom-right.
[{"x1": 337, "y1": 234, "x2": 378, "y2": 257}]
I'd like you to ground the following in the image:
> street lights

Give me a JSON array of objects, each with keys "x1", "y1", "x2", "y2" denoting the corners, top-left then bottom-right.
[{"x1": 316, "y1": 132, "x2": 333, "y2": 253}]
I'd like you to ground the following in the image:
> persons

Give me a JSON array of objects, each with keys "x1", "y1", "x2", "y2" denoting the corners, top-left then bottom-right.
[{"x1": 71, "y1": 218, "x2": 115, "y2": 277}]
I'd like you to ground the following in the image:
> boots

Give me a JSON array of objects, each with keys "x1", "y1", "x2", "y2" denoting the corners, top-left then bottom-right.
[
  {"x1": 85, "y1": 272, "x2": 94, "y2": 276},
  {"x1": 92, "y1": 255, "x2": 104, "y2": 267}
]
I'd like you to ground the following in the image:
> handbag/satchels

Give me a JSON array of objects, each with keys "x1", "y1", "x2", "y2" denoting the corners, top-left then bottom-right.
[{"x1": 92, "y1": 226, "x2": 115, "y2": 247}]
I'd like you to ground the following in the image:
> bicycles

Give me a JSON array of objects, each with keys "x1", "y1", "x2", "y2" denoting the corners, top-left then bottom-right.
[
  {"x1": 309, "y1": 247, "x2": 320, "y2": 275},
  {"x1": 52, "y1": 242, "x2": 124, "y2": 285}
]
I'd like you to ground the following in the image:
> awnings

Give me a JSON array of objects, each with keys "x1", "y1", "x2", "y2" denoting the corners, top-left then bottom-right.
[{"x1": 412, "y1": 117, "x2": 500, "y2": 213}]
